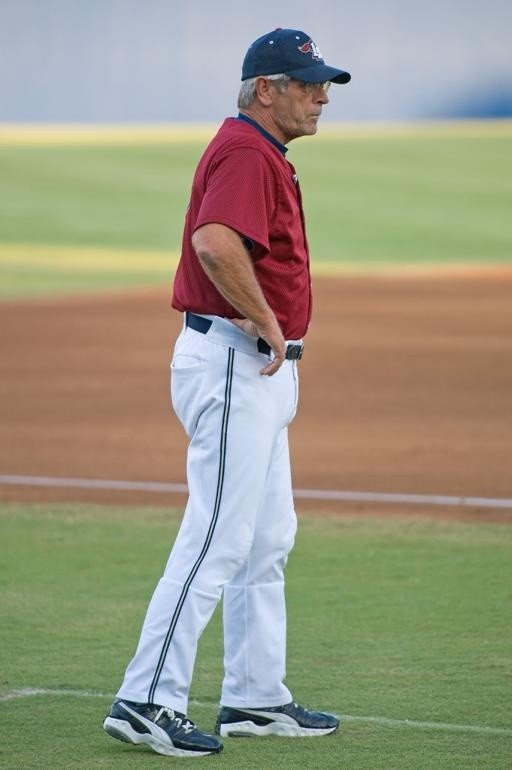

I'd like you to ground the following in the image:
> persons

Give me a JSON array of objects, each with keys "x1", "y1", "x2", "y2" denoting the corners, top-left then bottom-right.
[{"x1": 99, "y1": 26, "x2": 352, "y2": 759}]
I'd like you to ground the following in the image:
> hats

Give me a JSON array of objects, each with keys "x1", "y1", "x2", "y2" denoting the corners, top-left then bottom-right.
[{"x1": 241, "y1": 28, "x2": 351, "y2": 84}]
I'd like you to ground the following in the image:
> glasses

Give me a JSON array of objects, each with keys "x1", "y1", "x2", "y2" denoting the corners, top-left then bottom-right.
[{"x1": 306, "y1": 80, "x2": 330, "y2": 93}]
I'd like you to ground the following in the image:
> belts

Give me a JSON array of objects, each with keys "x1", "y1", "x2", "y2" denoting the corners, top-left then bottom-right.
[{"x1": 186, "y1": 312, "x2": 304, "y2": 360}]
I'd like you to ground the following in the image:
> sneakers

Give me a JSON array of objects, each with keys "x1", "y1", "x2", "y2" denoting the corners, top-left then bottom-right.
[
  {"x1": 102, "y1": 698, "x2": 223, "y2": 758},
  {"x1": 214, "y1": 702, "x2": 339, "y2": 737}
]
